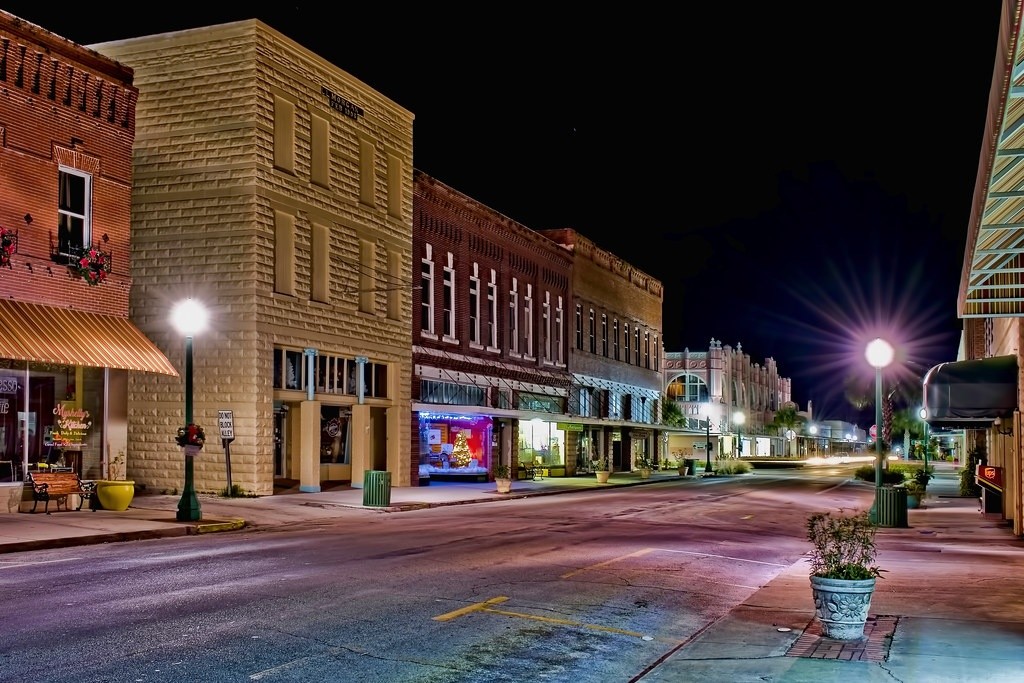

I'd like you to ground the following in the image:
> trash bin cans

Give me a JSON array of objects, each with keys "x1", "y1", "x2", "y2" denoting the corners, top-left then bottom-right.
[
  {"x1": 363, "y1": 470, "x2": 392, "y2": 507},
  {"x1": 876, "y1": 486, "x2": 908, "y2": 527},
  {"x1": 684, "y1": 459, "x2": 696, "y2": 475}
]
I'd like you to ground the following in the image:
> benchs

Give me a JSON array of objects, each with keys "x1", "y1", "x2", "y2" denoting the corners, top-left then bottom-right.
[
  {"x1": 522, "y1": 461, "x2": 544, "y2": 481},
  {"x1": 29, "y1": 473, "x2": 97, "y2": 515}
]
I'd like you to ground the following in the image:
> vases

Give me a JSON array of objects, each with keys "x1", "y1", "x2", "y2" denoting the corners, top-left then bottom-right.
[
  {"x1": 685, "y1": 458, "x2": 699, "y2": 476},
  {"x1": 185, "y1": 445, "x2": 201, "y2": 457}
]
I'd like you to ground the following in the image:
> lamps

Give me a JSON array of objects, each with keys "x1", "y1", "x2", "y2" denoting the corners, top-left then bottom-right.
[{"x1": 994, "y1": 417, "x2": 1012, "y2": 437}]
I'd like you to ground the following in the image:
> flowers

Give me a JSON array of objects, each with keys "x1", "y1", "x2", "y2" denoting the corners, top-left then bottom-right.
[
  {"x1": 0, "y1": 226, "x2": 19, "y2": 273},
  {"x1": 175, "y1": 422, "x2": 206, "y2": 449},
  {"x1": 74, "y1": 247, "x2": 111, "y2": 287}
]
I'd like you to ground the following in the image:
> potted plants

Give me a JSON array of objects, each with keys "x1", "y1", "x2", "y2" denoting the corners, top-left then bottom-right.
[
  {"x1": 492, "y1": 463, "x2": 512, "y2": 493},
  {"x1": 591, "y1": 457, "x2": 610, "y2": 483},
  {"x1": 903, "y1": 481, "x2": 924, "y2": 509},
  {"x1": 804, "y1": 507, "x2": 889, "y2": 641},
  {"x1": 672, "y1": 449, "x2": 689, "y2": 476},
  {"x1": 636, "y1": 451, "x2": 651, "y2": 479},
  {"x1": 94, "y1": 449, "x2": 135, "y2": 512}
]
